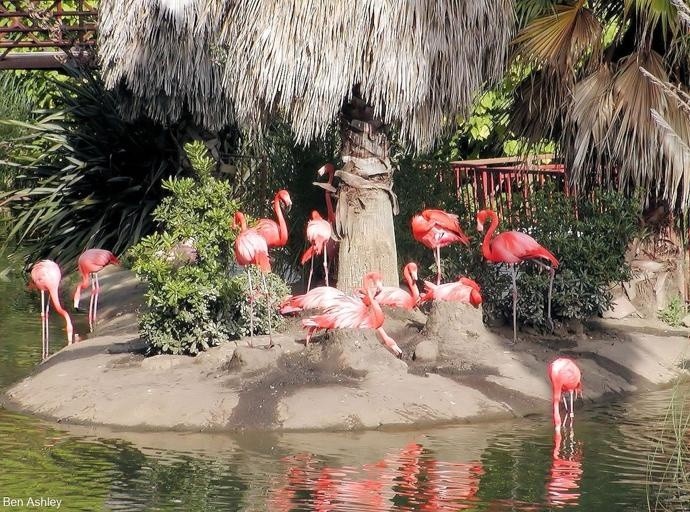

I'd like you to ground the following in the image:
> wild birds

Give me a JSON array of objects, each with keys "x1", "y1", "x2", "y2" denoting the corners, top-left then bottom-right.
[
  {"x1": 476, "y1": 208, "x2": 560, "y2": 346},
  {"x1": 27, "y1": 257, "x2": 77, "y2": 333},
  {"x1": 278, "y1": 271, "x2": 385, "y2": 347},
  {"x1": 228, "y1": 211, "x2": 277, "y2": 350},
  {"x1": 354, "y1": 261, "x2": 418, "y2": 360},
  {"x1": 418, "y1": 275, "x2": 483, "y2": 306},
  {"x1": 251, "y1": 190, "x2": 293, "y2": 249},
  {"x1": 300, "y1": 209, "x2": 334, "y2": 293},
  {"x1": 316, "y1": 160, "x2": 341, "y2": 224},
  {"x1": 409, "y1": 208, "x2": 471, "y2": 286},
  {"x1": 72, "y1": 248, "x2": 122, "y2": 325},
  {"x1": 547, "y1": 357, "x2": 582, "y2": 427}
]
